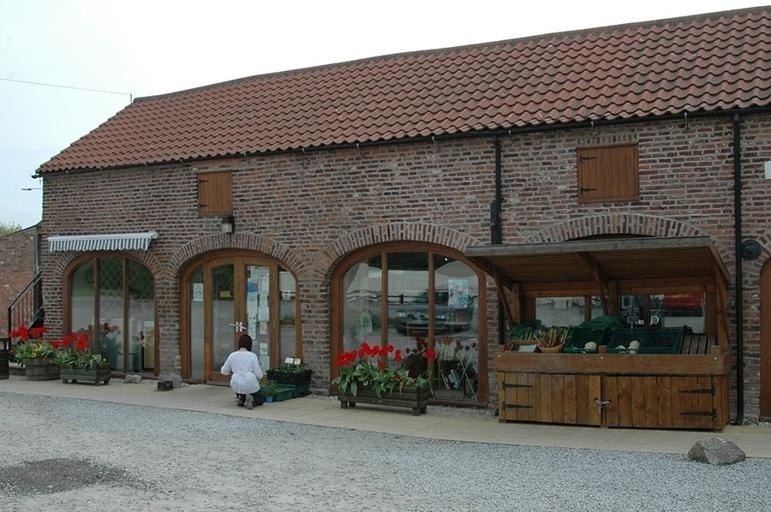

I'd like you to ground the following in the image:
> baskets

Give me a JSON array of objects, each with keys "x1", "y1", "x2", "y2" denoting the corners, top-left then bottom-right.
[
  {"x1": 512, "y1": 339, "x2": 537, "y2": 344},
  {"x1": 537, "y1": 342, "x2": 566, "y2": 353}
]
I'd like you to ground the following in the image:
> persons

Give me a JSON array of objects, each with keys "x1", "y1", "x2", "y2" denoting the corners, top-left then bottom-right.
[{"x1": 220, "y1": 334, "x2": 265, "y2": 409}]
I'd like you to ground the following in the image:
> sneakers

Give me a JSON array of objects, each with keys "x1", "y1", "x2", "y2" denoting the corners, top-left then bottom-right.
[
  {"x1": 237, "y1": 394, "x2": 246, "y2": 406},
  {"x1": 246, "y1": 395, "x2": 254, "y2": 409}
]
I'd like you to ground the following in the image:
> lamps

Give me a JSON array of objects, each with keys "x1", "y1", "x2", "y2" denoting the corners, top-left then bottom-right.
[{"x1": 220, "y1": 216, "x2": 235, "y2": 234}]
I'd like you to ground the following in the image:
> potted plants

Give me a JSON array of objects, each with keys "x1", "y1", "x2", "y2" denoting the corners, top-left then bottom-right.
[{"x1": 259, "y1": 365, "x2": 313, "y2": 403}]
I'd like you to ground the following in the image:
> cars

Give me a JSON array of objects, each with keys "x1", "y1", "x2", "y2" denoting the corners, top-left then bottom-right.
[{"x1": 395, "y1": 287, "x2": 474, "y2": 336}]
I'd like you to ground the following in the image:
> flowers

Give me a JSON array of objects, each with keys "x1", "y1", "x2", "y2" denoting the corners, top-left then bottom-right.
[
  {"x1": 7, "y1": 324, "x2": 111, "y2": 374},
  {"x1": 333, "y1": 341, "x2": 446, "y2": 401}
]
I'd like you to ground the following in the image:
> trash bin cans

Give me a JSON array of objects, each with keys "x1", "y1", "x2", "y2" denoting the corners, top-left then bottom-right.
[{"x1": 0, "y1": 338, "x2": 11, "y2": 379}]
